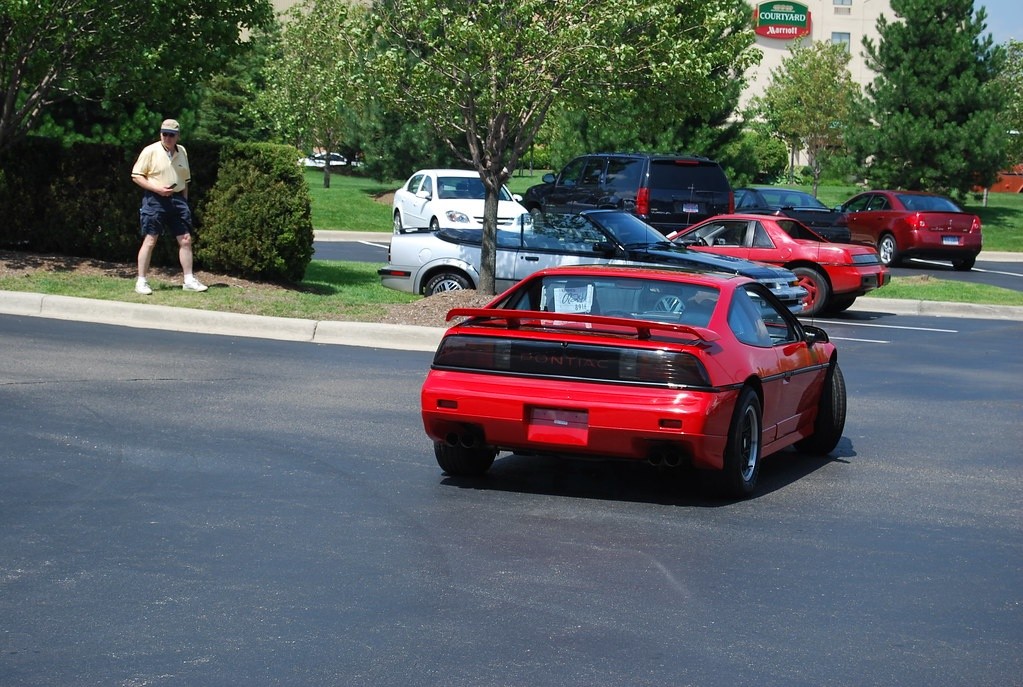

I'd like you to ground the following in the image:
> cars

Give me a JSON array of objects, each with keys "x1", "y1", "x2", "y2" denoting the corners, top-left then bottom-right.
[
  {"x1": 419, "y1": 261, "x2": 849, "y2": 504},
  {"x1": 731, "y1": 185, "x2": 851, "y2": 244},
  {"x1": 297, "y1": 152, "x2": 367, "y2": 168},
  {"x1": 664, "y1": 212, "x2": 893, "y2": 319},
  {"x1": 833, "y1": 189, "x2": 982, "y2": 271},
  {"x1": 390, "y1": 168, "x2": 535, "y2": 235}
]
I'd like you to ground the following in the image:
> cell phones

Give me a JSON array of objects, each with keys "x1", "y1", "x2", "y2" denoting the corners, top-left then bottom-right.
[{"x1": 167, "y1": 183, "x2": 177, "y2": 189}]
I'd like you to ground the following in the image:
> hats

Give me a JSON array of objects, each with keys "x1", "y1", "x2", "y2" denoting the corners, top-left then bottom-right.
[{"x1": 161, "y1": 119, "x2": 180, "y2": 135}]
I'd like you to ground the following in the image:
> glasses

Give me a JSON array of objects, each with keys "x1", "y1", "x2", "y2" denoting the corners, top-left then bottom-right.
[{"x1": 163, "y1": 132, "x2": 177, "y2": 138}]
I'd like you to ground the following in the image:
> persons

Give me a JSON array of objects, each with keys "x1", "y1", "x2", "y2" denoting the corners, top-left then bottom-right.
[{"x1": 131, "y1": 119, "x2": 209, "y2": 295}]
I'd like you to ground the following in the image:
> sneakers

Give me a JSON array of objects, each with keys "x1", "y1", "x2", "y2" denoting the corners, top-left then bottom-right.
[
  {"x1": 183, "y1": 280, "x2": 208, "y2": 292},
  {"x1": 135, "y1": 282, "x2": 152, "y2": 295}
]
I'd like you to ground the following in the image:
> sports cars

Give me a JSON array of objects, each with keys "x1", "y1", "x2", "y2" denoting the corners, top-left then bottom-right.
[{"x1": 375, "y1": 206, "x2": 809, "y2": 330}]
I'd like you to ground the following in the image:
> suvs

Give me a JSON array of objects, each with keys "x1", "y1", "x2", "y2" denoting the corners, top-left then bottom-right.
[{"x1": 522, "y1": 151, "x2": 735, "y2": 242}]
